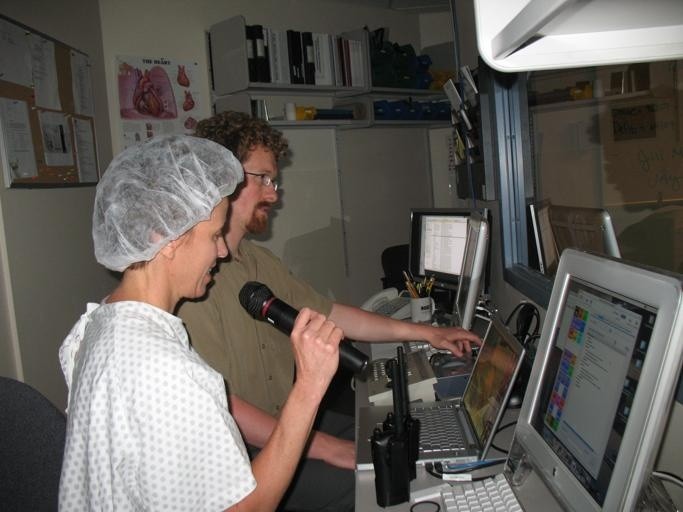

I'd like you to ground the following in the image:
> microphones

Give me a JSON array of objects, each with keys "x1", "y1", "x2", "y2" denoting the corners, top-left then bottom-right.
[{"x1": 237, "y1": 280, "x2": 370, "y2": 376}]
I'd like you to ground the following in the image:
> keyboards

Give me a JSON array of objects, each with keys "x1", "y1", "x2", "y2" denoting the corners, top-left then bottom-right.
[
  {"x1": 440, "y1": 473, "x2": 523, "y2": 512},
  {"x1": 403, "y1": 341, "x2": 452, "y2": 360}
]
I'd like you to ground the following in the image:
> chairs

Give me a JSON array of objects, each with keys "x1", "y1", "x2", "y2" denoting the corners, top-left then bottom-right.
[{"x1": 0, "y1": 373, "x2": 65, "y2": 512}]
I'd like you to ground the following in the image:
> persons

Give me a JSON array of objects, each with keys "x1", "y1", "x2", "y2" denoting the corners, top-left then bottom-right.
[
  {"x1": 59, "y1": 133, "x2": 346, "y2": 512},
  {"x1": 171, "y1": 108, "x2": 482, "y2": 511}
]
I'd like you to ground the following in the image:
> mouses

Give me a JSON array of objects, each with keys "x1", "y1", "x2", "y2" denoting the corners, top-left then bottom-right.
[{"x1": 429, "y1": 352, "x2": 450, "y2": 367}]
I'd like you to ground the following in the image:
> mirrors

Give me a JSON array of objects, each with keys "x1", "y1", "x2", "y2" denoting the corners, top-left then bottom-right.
[{"x1": 486, "y1": 58, "x2": 683, "y2": 405}]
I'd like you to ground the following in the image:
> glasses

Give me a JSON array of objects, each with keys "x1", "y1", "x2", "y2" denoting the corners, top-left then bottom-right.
[{"x1": 246, "y1": 171, "x2": 280, "y2": 191}]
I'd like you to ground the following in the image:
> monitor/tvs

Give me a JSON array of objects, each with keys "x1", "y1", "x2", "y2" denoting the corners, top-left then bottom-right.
[
  {"x1": 526, "y1": 198, "x2": 557, "y2": 275},
  {"x1": 455, "y1": 218, "x2": 488, "y2": 333},
  {"x1": 548, "y1": 205, "x2": 622, "y2": 262},
  {"x1": 408, "y1": 207, "x2": 492, "y2": 292},
  {"x1": 503, "y1": 247, "x2": 683, "y2": 512}
]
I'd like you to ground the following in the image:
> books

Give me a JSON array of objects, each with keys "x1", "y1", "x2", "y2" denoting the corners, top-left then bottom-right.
[
  {"x1": 242, "y1": 23, "x2": 391, "y2": 123},
  {"x1": 439, "y1": 65, "x2": 484, "y2": 168}
]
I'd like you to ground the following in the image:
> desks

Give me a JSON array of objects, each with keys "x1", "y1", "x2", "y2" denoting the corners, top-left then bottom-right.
[{"x1": 353, "y1": 293, "x2": 637, "y2": 512}]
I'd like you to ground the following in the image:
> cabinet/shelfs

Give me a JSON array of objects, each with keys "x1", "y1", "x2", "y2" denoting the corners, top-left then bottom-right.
[{"x1": 210, "y1": 14, "x2": 451, "y2": 130}]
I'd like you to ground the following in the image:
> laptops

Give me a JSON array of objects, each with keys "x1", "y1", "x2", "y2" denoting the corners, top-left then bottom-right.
[{"x1": 355, "y1": 317, "x2": 527, "y2": 472}]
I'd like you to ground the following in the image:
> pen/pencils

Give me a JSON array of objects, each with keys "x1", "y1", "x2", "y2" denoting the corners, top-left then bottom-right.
[{"x1": 403, "y1": 270, "x2": 436, "y2": 298}]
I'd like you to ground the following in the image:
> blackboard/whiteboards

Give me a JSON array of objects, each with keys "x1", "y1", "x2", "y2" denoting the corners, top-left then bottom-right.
[{"x1": 0, "y1": 13, "x2": 101, "y2": 189}]
